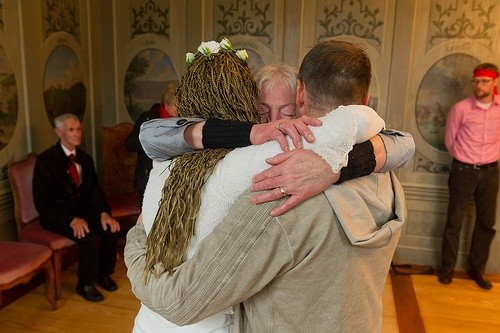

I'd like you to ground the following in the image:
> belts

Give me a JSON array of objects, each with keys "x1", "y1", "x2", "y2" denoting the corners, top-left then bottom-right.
[{"x1": 452, "y1": 158, "x2": 497, "y2": 170}]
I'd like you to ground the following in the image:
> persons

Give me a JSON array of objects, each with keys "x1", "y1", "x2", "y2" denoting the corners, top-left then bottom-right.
[
  {"x1": 32, "y1": 114, "x2": 121, "y2": 302},
  {"x1": 438, "y1": 63, "x2": 500, "y2": 290},
  {"x1": 124, "y1": 32, "x2": 417, "y2": 333}
]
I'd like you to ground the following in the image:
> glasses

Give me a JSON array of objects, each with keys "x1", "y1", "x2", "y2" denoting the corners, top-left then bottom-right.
[{"x1": 469, "y1": 79, "x2": 495, "y2": 85}]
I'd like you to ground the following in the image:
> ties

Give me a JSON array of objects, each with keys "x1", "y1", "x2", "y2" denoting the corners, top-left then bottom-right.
[{"x1": 69, "y1": 154, "x2": 79, "y2": 189}]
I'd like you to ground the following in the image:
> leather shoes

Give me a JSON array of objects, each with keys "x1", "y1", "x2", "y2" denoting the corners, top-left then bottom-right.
[
  {"x1": 96, "y1": 273, "x2": 118, "y2": 291},
  {"x1": 76, "y1": 279, "x2": 103, "y2": 301}
]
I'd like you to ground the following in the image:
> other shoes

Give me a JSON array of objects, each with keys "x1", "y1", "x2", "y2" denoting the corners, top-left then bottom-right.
[
  {"x1": 472, "y1": 269, "x2": 492, "y2": 289},
  {"x1": 439, "y1": 268, "x2": 452, "y2": 284}
]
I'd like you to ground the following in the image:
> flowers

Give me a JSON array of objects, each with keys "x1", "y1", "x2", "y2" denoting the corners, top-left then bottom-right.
[{"x1": 186, "y1": 37, "x2": 249, "y2": 64}]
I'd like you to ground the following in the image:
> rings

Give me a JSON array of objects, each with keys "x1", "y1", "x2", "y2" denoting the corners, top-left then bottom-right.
[
  {"x1": 279, "y1": 186, "x2": 287, "y2": 196},
  {"x1": 277, "y1": 121, "x2": 285, "y2": 129}
]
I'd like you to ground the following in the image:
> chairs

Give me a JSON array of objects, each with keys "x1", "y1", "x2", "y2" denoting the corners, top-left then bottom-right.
[{"x1": 0, "y1": 121, "x2": 142, "y2": 310}]
